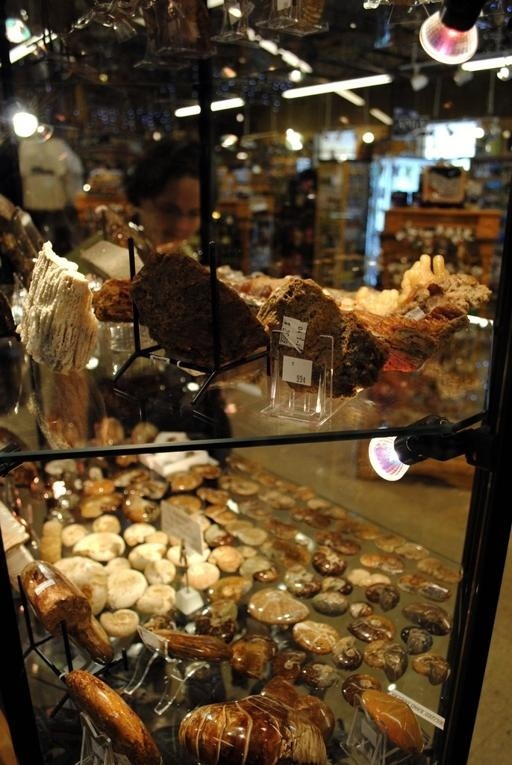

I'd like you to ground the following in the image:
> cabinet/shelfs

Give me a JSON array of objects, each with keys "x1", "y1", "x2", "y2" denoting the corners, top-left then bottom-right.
[{"x1": 311, "y1": 162, "x2": 367, "y2": 288}]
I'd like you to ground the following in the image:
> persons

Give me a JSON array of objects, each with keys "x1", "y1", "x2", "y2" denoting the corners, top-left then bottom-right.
[{"x1": 56, "y1": 139, "x2": 220, "y2": 278}]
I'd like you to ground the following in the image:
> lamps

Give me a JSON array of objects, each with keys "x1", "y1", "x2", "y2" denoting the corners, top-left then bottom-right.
[{"x1": 418, "y1": 1, "x2": 483, "y2": 65}]
